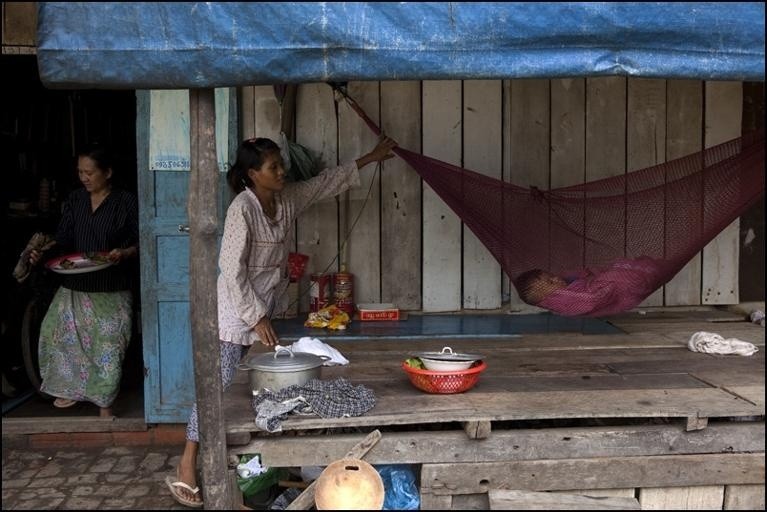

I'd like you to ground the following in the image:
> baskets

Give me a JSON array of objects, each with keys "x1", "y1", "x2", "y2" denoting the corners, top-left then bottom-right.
[{"x1": 401, "y1": 357, "x2": 487, "y2": 394}]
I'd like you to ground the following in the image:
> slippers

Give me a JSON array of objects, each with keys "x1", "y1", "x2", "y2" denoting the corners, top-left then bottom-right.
[
  {"x1": 165, "y1": 475, "x2": 205, "y2": 508},
  {"x1": 50, "y1": 396, "x2": 78, "y2": 409}
]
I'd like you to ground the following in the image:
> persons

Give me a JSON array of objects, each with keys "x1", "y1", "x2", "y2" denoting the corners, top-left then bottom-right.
[
  {"x1": 23, "y1": 138, "x2": 139, "y2": 419},
  {"x1": 162, "y1": 133, "x2": 398, "y2": 507},
  {"x1": 514, "y1": 235, "x2": 694, "y2": 319}
]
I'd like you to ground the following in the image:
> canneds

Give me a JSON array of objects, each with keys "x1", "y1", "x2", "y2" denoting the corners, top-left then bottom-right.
[
  {"x1": 311, "y1": 273, "x2": 331, "y2": 312},
  {"x1": 333, "y1": 272, "x2": 354, "y2": 319}
]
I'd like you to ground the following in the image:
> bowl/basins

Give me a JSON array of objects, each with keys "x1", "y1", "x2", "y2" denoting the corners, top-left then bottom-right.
[{"x1": 416, "y1": 357, "x2": 474, "y2": 373}]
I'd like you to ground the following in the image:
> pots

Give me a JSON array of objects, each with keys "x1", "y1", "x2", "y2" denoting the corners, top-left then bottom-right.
[{"x1": 233, "y1": 347, "x2": 335, "y2": 401}]
